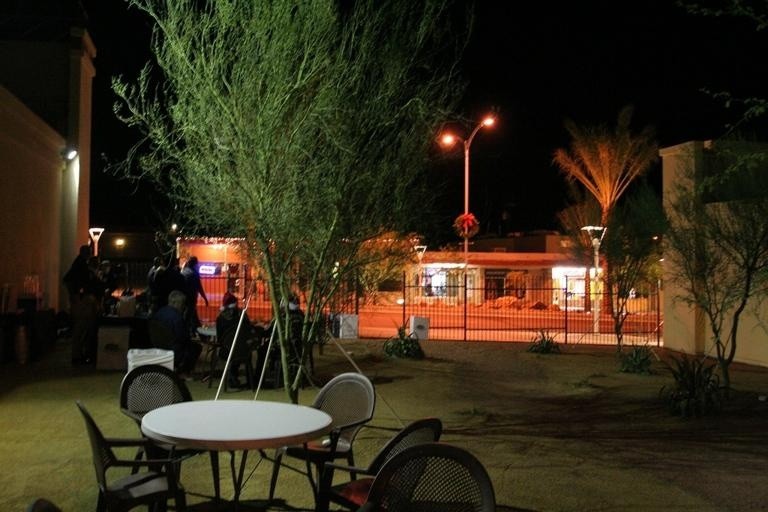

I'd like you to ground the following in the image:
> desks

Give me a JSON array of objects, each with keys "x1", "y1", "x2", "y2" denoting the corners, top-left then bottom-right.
[
  {"x1": 141, "y1": 397, "x2": 333, "y2": 512},
  {"x1": 198, "y1": 326, "x2": 263, "y2": 383}
]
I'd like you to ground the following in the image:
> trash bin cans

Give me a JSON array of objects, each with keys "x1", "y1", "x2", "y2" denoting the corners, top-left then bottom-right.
[
  {"x1": 127, "y1": 349, "x2": 173, "y2": 412},
  {"x1": 410, "y1": 318, "x2": 429, "y2": 339},
  {"x1": 340, "y1": 314, "x2": 359, "y2": 340}
]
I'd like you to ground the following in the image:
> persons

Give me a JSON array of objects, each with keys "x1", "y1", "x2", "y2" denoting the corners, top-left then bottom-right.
[
  {"x1": 63, "y1": 242, "x2": 211, "y2": 353},
  {"x1": 257, "y1": 293, "x2": 305, "y2": 368},
  {"x1": 425, "y1": 284, "x2": 434, "y2": 296},
  {"x1": 215, "y1": 293, "x2": 253, "y2": 388},
  {"x1": 150, "y1": 290, "x2": 203, "y2": 381}
]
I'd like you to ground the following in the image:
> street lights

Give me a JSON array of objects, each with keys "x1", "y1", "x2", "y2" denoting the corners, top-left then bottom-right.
[{"x1": 438, "y1": 114, "x2": 498, "y2": 262}]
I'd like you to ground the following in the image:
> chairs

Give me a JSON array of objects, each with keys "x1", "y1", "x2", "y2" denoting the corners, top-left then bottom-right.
[
  {"x1": 359, "y1": 442, "x2": 496, "y2": 512},
  {"x1": 269, "y1": 373, "x2": 376, "y2": 510},
  {"x1": 119, "y1": 364, "x2": 220, "y2": 502},
  {"x1": 263, "y1": 315, "x2": 316, "y2": 392},
  {"x1": 209, "y1": 324, "x2": 256, "y2": 392},
  {"x1": 320, "y1": 417, "x2": 442, "y2": 510},
  {"x1": 75, "y1": 400, "x2": 188, "y2": 512}
]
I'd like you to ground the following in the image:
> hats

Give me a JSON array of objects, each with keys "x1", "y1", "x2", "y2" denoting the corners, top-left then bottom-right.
[
  {"x1": 280, "y1": 295, "x2": 300, "y2": 311},
  {"x1": 219, "y1": 292, "x2": 238, "y2": 312}
]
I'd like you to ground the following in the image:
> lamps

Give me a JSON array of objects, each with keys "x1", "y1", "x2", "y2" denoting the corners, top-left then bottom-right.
[{"x1": 60, "y1": 145, "x2": 78, "y2": 160}]
